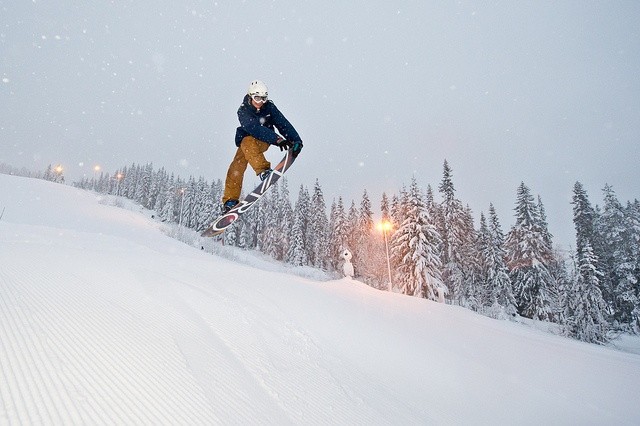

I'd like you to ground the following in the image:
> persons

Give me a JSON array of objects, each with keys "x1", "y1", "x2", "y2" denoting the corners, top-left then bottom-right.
[{"x1": 221, "y1": 81, "x2": 302, "y2": 215}]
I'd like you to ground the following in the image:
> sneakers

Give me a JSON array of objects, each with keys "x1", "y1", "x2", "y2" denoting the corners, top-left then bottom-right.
[
  {"x1": 219, "y1": 199, "x2": 239, "y2": 214},
  {"x1": 258, "y1": 167, "x2": 273, "y2": 180}
]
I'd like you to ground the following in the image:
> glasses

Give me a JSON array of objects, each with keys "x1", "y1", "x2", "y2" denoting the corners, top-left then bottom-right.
[{"x1": 251, "y1": 94, "x2": 267, "y2": 102}]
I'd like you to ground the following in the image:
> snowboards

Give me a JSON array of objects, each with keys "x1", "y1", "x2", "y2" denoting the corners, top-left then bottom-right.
[{"x1": 201, "y1": 146, "x2": 298, "y2": 237}]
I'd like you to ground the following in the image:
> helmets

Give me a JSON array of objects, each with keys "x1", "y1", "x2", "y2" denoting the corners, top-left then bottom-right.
[{"x1": 248, "y1": 79, "x2": 267, "y2": 96}]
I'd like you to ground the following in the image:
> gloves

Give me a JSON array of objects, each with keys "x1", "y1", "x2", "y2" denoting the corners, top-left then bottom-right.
[
  {"x1": 275, "y1": 136, "x2": 292, "y2": 150},
  {"x1": 293, "y1": 141, "x2": 303, "y2": 156}
]
